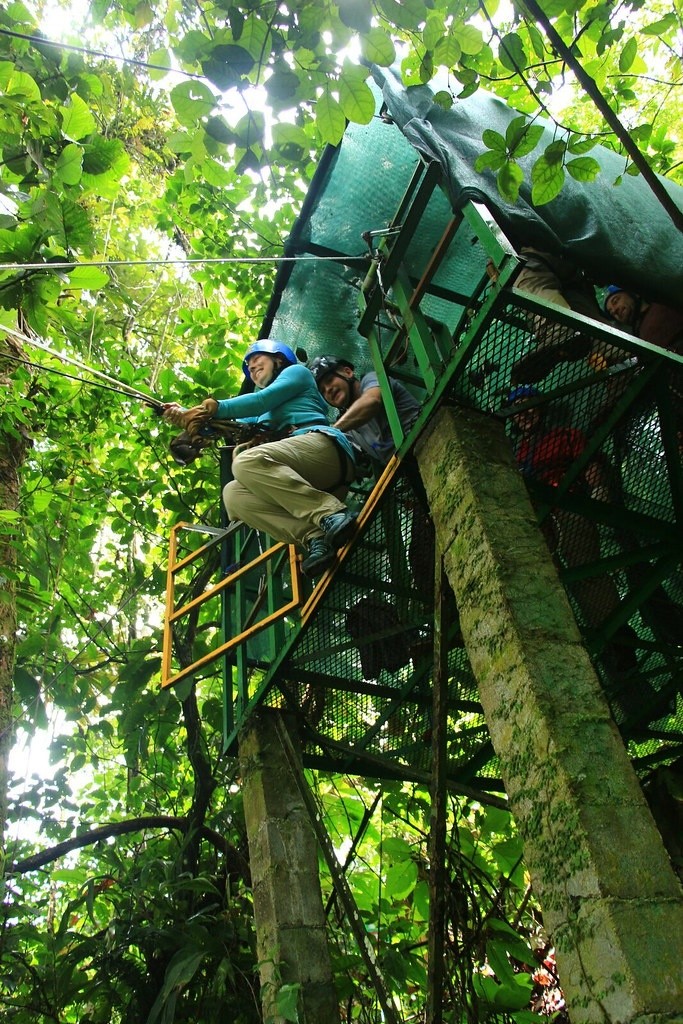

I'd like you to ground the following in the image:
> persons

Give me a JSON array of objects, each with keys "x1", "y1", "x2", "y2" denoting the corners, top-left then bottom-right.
[
  {"x1": 486, "y1": 239, "x2": 607, "y2": 385},
  {"x1": 602, "y1": 283, "x2": 683, "y2": 390},
  {"x1": 161, "y1": 338, "x2": 357, "y2": 579},
  {"x1": 309, "y1": 354, "x2": 465, "y2": 646},
  {"x1": 505, "y1": 386, "x2": 682, "y2": 721}
]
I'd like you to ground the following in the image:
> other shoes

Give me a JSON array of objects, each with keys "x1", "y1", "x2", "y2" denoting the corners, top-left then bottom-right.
[{"x1": 510, "y1": 331, "x2": 590, "y2": 383}]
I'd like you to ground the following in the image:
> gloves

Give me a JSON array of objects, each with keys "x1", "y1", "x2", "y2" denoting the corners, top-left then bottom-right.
[{"x1": 177, "y1": 398, "x2": 219, "y2": 434}]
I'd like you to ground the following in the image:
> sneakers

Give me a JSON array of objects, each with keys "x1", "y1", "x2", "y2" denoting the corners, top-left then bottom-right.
[{"x1": 300, "y1": 509, "x2": 361, "y2": 579}]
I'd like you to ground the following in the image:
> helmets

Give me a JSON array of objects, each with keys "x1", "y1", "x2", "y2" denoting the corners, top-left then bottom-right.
[
  {"x1": 310, "y1": 354, "x2": 354, "y2": 391},
  {"x1": 242, "y1": 339, "x2": 297, "y2": 380},
  {"x1": 604, "y1": 285, "x2": 622, "y2": 303},
  {"x1": 505, "y1": 386, "x2": 543, "y2": 405}
]
